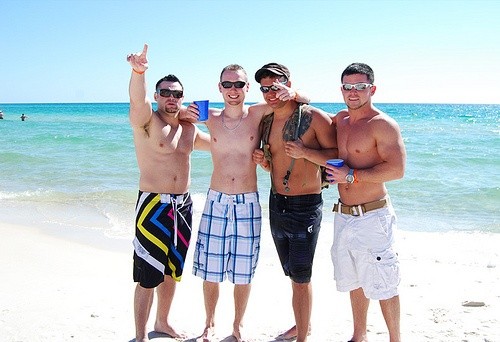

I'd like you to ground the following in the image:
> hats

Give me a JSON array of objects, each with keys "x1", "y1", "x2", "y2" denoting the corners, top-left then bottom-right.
[{"x1": 254, "y1": 62, "x2": 290, "y2": 83}]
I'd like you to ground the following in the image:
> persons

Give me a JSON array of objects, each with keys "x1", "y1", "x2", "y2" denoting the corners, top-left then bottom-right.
[
  {"x1": 124, "y1": 41, "x2": 211, "y2": 342},
  {"x1": 249, "y1": 62, "x2": 338, "y2": 342},
  {"x1": 20, "y1": 113, "x2": 26, "y2": 121},
  {"x1": 178, "y1": 64, "x2": 312, "y2": 342},
  {"x1": 0, "y1": 111, "x2": 4, "y2": 119},
  {"x1": 322, "y1": 61, "x2": 406, "y2": 342}
]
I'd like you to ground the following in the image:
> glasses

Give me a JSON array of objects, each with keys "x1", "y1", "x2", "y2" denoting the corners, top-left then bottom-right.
[
  {"x1": 157, "y1": 89, "x2": 183, "y2": 98},
  {"x1": 342, "y1": 83, "x2": 371, "y2": 90},
  {"x1": 260, "y1": 82, "x2": 286, "y2": 92},
  {"x1": 221, "y1": 80, "x2": 246, "y2": 88}
]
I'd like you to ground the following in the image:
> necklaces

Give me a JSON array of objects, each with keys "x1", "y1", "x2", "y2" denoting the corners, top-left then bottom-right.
[{"x1": 221, "y1": 108, "x2": 244, "y2": 131}]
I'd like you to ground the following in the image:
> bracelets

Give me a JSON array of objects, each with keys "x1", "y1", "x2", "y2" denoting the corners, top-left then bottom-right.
[
  {"x1": 132, "y1": 68, "x2": 145, "y2": 75},
  {"x1": 294, "y1": 89, "x2": 298, "y2": 100},
  {"x1": 353, "y1": 169, "x2": 359, "y2": 184}
]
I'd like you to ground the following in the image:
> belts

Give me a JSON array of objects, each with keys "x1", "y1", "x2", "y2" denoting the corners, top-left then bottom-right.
[{"x1": 333, "y1": 199, "x2": 385, "y2": 216}]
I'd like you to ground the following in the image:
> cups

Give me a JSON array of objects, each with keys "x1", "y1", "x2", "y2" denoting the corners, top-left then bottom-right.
[
  {"x1": 193, "y1": 100, "x2": 209, "y2": 121},
  {"x1": 326, "y1": 159, "x2": 344, "y2": 182}
]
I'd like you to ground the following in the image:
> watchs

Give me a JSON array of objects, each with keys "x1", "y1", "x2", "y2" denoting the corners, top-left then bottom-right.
[{"x1": 345, "y1": 169, "x2": 354, "y2": 184}]
